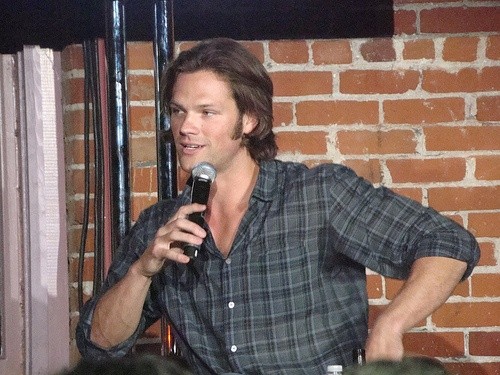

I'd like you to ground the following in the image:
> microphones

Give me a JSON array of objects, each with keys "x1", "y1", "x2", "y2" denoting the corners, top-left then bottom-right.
[{"x1": 183, "y1": 161, "x2": 217, "y2": 258}]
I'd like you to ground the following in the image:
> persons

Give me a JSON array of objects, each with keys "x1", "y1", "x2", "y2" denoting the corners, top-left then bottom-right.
[
  {"x1": 53, "y1": 353, "x2": 449, "y2": 375},
  {"x1": 74, "y1": 39, "x2": 483, "y2": 365}
]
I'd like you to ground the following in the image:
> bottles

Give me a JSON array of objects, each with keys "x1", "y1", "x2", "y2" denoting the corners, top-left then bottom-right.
[{"x1": 327, "y1": 365, "x2": 343, "y2": 375}]
